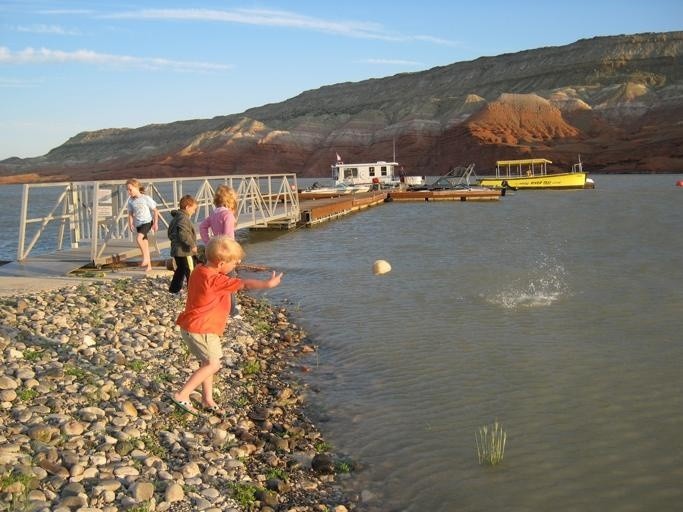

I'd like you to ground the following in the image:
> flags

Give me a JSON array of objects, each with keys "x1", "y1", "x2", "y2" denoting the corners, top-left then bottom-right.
[{"x1": 335, "y1": 154, "x2": 341, "y2": 161}]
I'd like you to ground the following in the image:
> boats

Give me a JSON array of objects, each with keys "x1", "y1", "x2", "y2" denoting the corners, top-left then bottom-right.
[
  {"x1": 476, "y1": 153, "x2": 596, "y2": 191},
  {"x1": 292, "y1": 136, "x2": 427, "y2": 193}
]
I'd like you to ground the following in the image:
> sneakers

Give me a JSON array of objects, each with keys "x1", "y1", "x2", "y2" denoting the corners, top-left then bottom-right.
[{"x1": 233, "y1": 314, "x2": 242, "y2": 319}]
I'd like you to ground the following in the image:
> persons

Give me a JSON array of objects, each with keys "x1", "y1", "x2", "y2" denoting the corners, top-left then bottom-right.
[
  {"x1": 397, "y1": 165, "x2": 406, "y2": 184},
  {"x1": 123, "y1": 177, "x2": 159, "y2": 274},
  {"x1": 198, "y1": 184, "x2": 243, "y2": 320},
  {"x1": 165, "y1": 193, "x2": 198, "y2": 295},
  {"x1": 166, "y1": 232, "x2": 283, "y2": 419}
]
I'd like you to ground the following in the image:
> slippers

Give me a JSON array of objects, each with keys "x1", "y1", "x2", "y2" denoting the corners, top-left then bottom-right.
[
  {"x1": 204, "y1": 405, "x2": 223, "y2": 416},
  {"x1": 171, "y1": 395, "x2": 197, "y2": 416}
]
